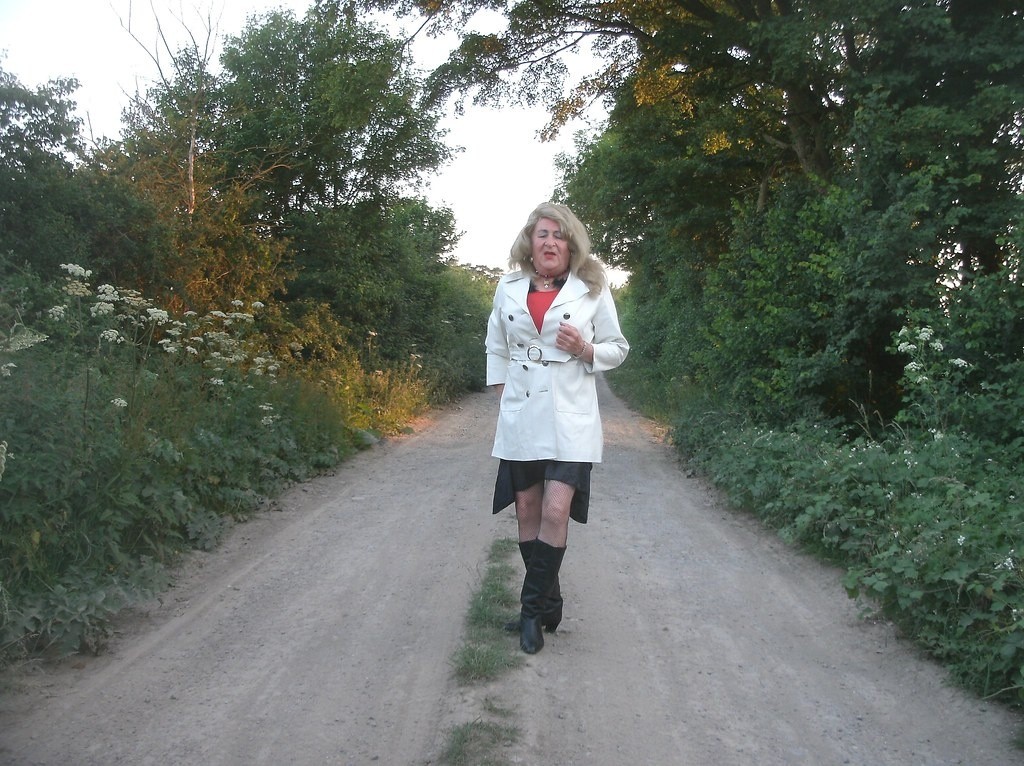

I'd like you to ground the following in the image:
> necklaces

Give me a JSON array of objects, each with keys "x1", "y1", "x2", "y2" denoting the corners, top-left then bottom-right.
[{"x1": 534, "y1": 265, "x2": 570, "y2": 288}]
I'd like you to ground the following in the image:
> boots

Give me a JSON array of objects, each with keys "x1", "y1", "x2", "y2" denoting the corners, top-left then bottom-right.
[
  {"x1": 505, "y1": 537, "x2": 563, "y2": 633},
  {"x1": 519, "y1": 536, "x2": 569, "y2": 654}
]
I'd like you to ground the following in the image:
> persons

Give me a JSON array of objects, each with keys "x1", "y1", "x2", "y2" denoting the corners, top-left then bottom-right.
[{"x1": 484, "y1": 202, "x2": 630, "y2": 655}]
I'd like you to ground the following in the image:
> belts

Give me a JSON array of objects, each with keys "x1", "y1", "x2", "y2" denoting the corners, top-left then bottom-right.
[{"x1": 509, "y1": 346, "x2": 574, "y2": 362}]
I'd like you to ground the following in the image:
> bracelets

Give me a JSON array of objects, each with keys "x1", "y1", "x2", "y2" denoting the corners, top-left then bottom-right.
[{"x1": 572, "y1": 340, "x2": 587, "y2": 359}]
